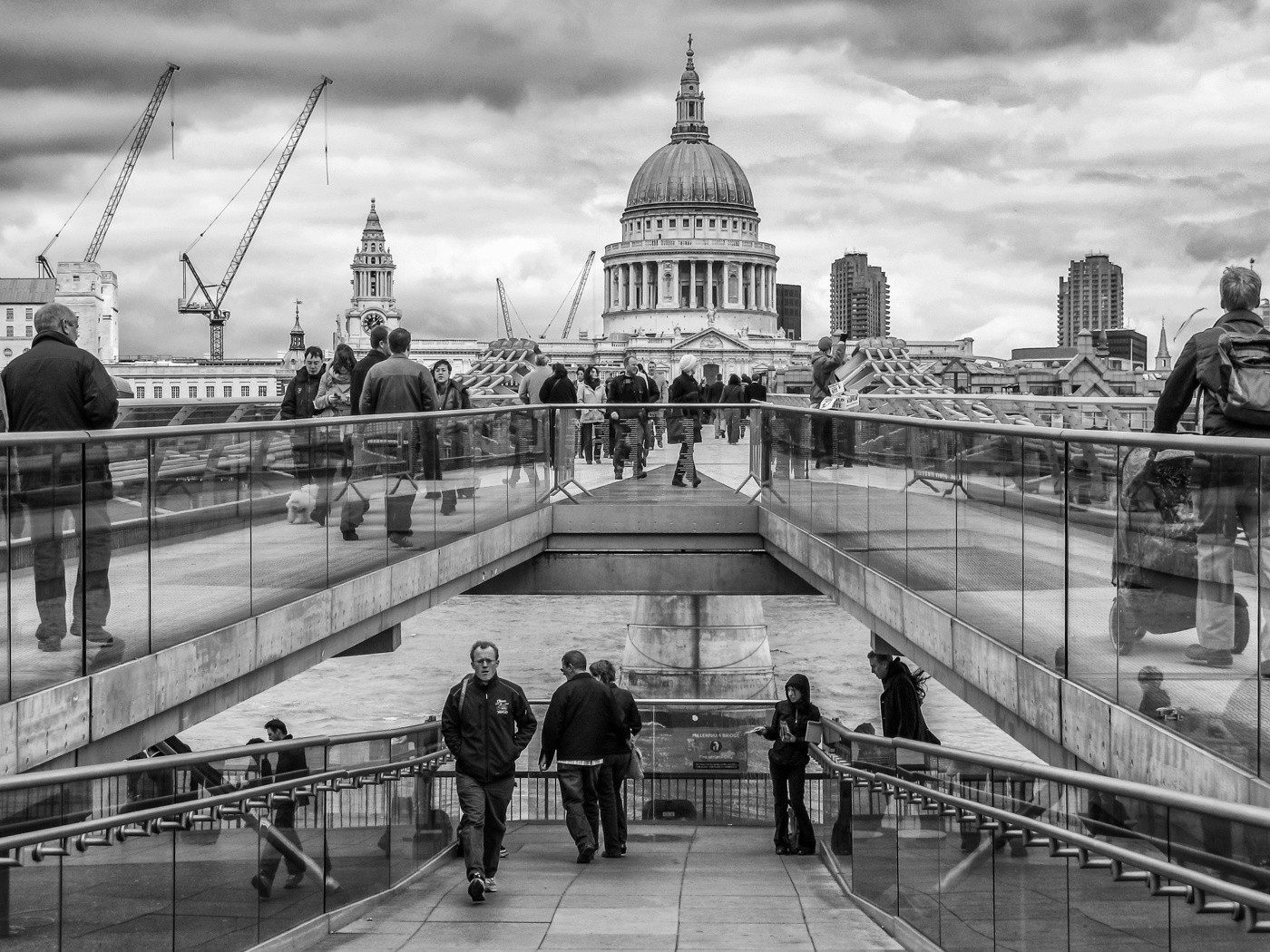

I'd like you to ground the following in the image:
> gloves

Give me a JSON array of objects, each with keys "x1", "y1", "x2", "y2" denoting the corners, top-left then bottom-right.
[{"x1": 839, "y1": 333, "x2": 848, "y2": 342}]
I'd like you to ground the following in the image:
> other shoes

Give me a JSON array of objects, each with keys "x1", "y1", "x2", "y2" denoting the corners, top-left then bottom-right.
[
  {"x1": 775, "y1": 845, "x2": 815, "y2": 855},
  {"x1": 424, "y1": 483, "x2": 474, "y2": 515},
  {"x1": 671, "y1": 478, "x2": 701, "y2": 488},
  {"x1": 576, "y1": 843, "x2": 627, "y2": 865},
  {"x1": 548, "y1": 439, "x2": 663, "y2": 480},
  {"x1": 340, "y1": 521, "x2": 359, "y2": 541},
  {"x1": 310, "y1": 509, "x2": 325, "y2": 527},
  {"x1": 38, "y1": 622, "x2": 114, "y2": 652},
  {"x1": 387, "y1": 529, "x2": 415, "y2": 547},
  {"x1": 714, "y1": 429, "x2": 744, "y2": 446},
  {"x1": 502, "y1": 478, "x2": 540, "y2": 489},
  {"x1": 499, "y1": 845, "x2": 508, "y2": 857},
  {"x1": 772, "y1": 469, "x2": 806, "y2": 481}
]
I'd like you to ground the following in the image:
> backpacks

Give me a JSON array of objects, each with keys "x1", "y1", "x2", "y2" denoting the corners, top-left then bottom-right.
[{"x1": 1207, "y1": 323, "x2": 1270, "y2": 425}]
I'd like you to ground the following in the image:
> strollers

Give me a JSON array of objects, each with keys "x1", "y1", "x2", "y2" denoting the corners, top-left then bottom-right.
[{"x1": 1109, "y1": 427, "x2": 1250, "y2": 663}]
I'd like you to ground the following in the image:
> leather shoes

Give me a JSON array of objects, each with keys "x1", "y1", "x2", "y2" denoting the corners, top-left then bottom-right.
[{"x1": 1184, "y1": 644, "x2": 1233, "y2": 666}]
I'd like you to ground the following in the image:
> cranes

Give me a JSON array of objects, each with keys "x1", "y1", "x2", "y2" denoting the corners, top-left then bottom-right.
[
  {"x1": 30, "y1": 60, "x2": 181, "y2": 285},
  {"x1": 537, "y1": 252, "x2": 597, "y2": 339},
  {"x1": 174, "y1": 74, "x2": 336, "y2": 368},
  {"x1": 494, "y1": 276, "x2": 532, "y2": 340}
]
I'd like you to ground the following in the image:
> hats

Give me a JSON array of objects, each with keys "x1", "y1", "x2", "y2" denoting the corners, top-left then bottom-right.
[
  {"x1": 679, "y1": 354, "x2": 697, "y2": 372},
  {"x1": 818, "y1": 336, "x2": 833, "y2": 351}
]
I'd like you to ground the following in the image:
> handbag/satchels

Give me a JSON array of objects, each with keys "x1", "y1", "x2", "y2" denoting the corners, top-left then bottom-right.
[
  {"x1": 786, "y1": 803, "x2": 801, "y2": 855},
  {"x1": 625, "y1": 740, "x2": 646, "y2": 780},
  {"x1": 663, "y1": 399, "x2": 684, "y2": 419}
]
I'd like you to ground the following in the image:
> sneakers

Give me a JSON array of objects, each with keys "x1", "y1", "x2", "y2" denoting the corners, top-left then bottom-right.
[
  {"x1": 250, "y1": 871, "x2": 304, "y2": 897},
  {"x1": 467, "y1": 878, "x2": 498, "y2": 901}
]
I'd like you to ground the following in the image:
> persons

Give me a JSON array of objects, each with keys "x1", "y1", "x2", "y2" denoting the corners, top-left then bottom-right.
[
  {"x1": 805, "y1": 330, "x2": 848, "y2": 468},
  {"x1": 337, "y1": 328, "x2": 440, "y2": 552},
  {"x1": 502, "y1": 411, "x2": 542, "y2": 489},
  {"x1": 517, "y1": 354, "x2": 551, "y2": 443},
  {"x1": 312, "y1": 340, "x2": 361, "y2": 442},
  {"x1": 1150, "y1": 264, "x2": 1270, "y2": 676},
  {"x1": 348, "y1": 323, "x2": 393, "y2": 418},
  {"x1": 540, "y1": 649, "x2": 629, "y2": 864},
  {"x1": 573, "y1": 351, "x2": 769, "y2": 485},
  {"x1": 861, "y1": 649, "x2": 940, "y2": 747},
  {"x1": 1132, "y1": 664, "x2": 1171, "y2": 716},
  {"x1": 427, "y1": 356, "x2": 480, "y2": 516},
  {"x1": 277, "y1": 343, "x2": 327, "y2": 489},
  {"x1": 749, "y1": 671, "x2": 827, "y2": 859},
  {"x1": 439, "y1": 637, "x2": 539, "y2": 905},
  {"x1": 584, "y1": 659, "x2": 645, "y2": 859},
  {"x1": 537, "y1": 362, "x2": 577, "y2": 466},
  {"x1": 0, "y1": 305, "x2": 134, "y2": 654},
  {"x1": 249, "y1": 717, "x2": 308, "y2": 901}
]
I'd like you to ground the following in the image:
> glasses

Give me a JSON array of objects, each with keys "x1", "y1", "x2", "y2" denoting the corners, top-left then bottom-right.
[{"x1": 560, "y1": 668, "x2": 570, "y2": 672}]
